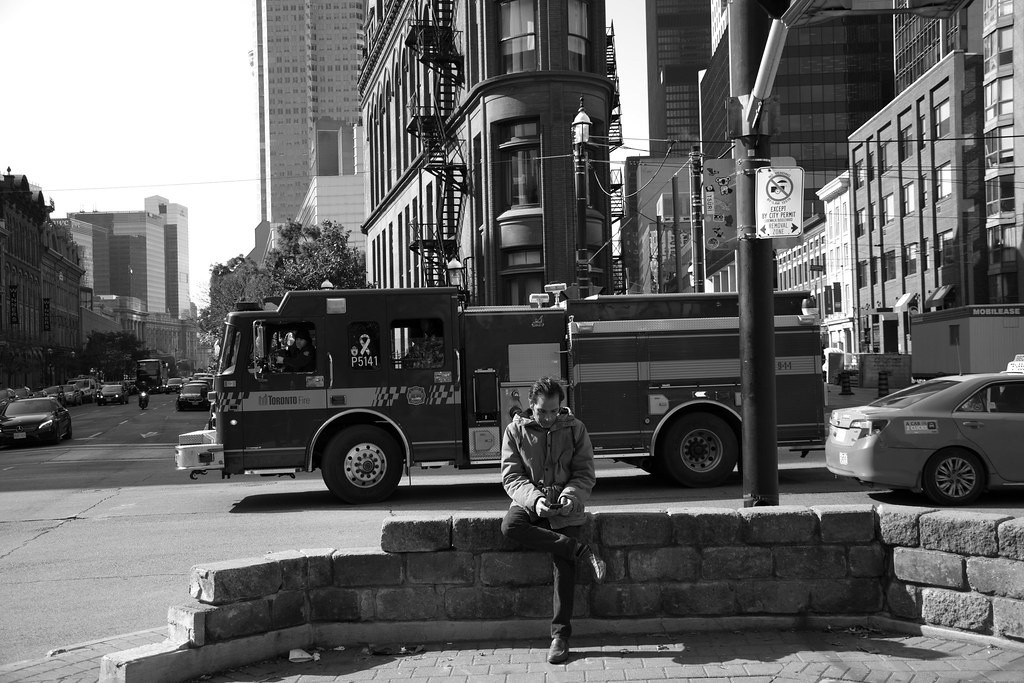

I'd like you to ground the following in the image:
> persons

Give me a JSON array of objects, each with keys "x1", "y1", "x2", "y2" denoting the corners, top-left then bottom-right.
[
  {"x1": 279, "y1": 329, "x2": 315, "y2": 375},
  {"x1": 137, "y1": 382, "x2": 150, "y2": 407},
  {"x1": 501, "y1": 376, "x2": 607, "y2": 663}
]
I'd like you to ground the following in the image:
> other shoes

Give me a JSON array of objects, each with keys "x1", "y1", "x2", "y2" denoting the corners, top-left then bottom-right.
[
  {"x1": 547, "y1": 631, "x2": 570, "y2": 667},
  {"x1": 581, "y1": 546, "x2": 608, "y2": 586}
]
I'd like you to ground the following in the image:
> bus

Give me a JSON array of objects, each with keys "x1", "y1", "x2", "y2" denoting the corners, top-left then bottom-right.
[{"x1": 136, "y1": 359, "x2": 165, "y2": 394}]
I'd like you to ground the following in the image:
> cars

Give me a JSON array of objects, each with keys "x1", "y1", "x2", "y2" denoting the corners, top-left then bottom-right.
[
  {"x1": 177, "y1": 372, "x2": 213, "y2": 411},
  {"x1": 98, "y1": 384, "x2": 127, "y2": 405},
  {"x1": 166, "y1": 377, "x2": 184, "y2": 393},
  {"x1": 0, "y1": 397, "x2": 73, "y2": 449},
  {"x1": 0, "y1": 382, "x2": 60, "y2": 420},
  {"x1": 824, "y1": 353, "x2": 1024, "y2": 506},
  {"x1": 62, "y1": 373, "x2": 136, "y2": 405}
]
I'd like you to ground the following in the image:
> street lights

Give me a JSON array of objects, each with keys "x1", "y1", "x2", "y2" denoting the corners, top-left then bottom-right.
[{"x1": 572, "y1": 94, "x2": 594, "y2": 298}]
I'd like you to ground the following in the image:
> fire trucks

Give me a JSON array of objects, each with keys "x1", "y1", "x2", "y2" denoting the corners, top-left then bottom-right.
[{"x1": 174, "y1": 279, "x2": 824, "y2": 506}]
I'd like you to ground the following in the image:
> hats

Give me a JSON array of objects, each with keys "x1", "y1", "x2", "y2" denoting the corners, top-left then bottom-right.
[{"x1": 294, "y1": 329, "x2": 310, "y2": 341}]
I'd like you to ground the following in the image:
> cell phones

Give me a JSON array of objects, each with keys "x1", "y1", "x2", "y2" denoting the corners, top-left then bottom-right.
[{"x1": 549, "y1": 503, "x2": 564, "y2": 509}]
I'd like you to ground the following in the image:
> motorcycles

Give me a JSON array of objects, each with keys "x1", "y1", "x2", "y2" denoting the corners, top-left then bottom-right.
[{"x1": 137, "y1": 387, "x2": 152, "y2": 410}]
